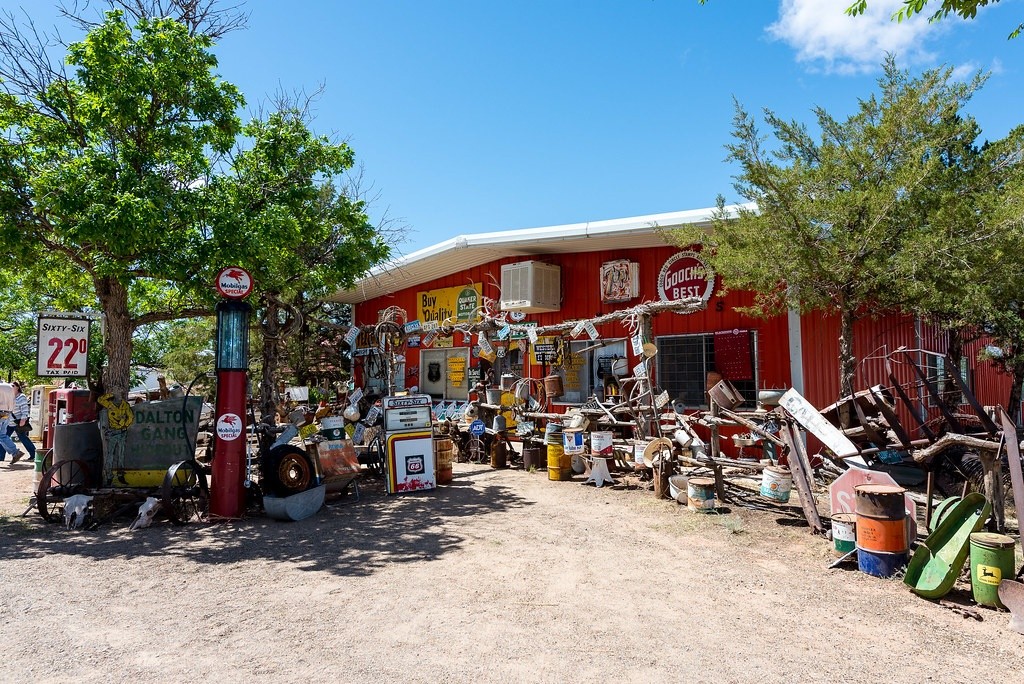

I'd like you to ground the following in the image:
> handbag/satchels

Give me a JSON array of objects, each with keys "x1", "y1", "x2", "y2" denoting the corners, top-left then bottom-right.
[{"x1": 13, "y1": 417, "x2": 33, "y2": 432}]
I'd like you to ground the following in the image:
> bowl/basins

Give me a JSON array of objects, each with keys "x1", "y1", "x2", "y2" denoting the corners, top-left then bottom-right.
[{"x1": 642, "y1": 437, "x2": 674, "y2": 467}]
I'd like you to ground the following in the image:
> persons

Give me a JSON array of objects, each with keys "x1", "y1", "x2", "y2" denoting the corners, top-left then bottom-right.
[
  {"x1": 0, "y1": 380, "x2": 37, "y2": 462},
  {"x1": 0, "y1": 375, "x2": 25, "y2": 465}
]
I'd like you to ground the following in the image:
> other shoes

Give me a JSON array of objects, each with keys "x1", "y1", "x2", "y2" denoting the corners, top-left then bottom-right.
[
  {"x1": 10, "y1": 451, "x2": 25, "y2": 464},
  {"x1": 28, "y1": 456, "x2": 35, "y2": 460}
]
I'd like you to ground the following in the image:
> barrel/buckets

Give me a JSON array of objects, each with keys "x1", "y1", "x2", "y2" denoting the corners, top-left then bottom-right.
[
  {"x1": 35, "y1": 448, "x2": 51, "y2": 495},
  {"x1": 486, "y1": 386, "x2": 503, "y2": 405},
  {"x1": 433, "y1": 435, "x2": 452, "y2": 484},
  {"x1": 760, "y1": 466, "x2": 792, "y2": 503},
  {"x1": 563, "y1": 427, "x2": 584, "y2": 455},
  {"x1": 523, "y1": 448, "x2": 540, "y2": 469},
  {"x1": 547, "y1": 432, "x2": 571, "y2": 480},
  {"x1": 55, "y1": 420, "x2": 103, "y2": 488},
  {"x1": 686, "y1": 477, "x2": 716, "y2": 512},
  {"x1": 971, "y1": 531, "x2": 1016, "y2": 608},
  {"x1": 855, "y1": 482, "x2": 908, "y2": 577},
  {"x1": 322, "y1": 416, "x2": 345, "y2": 439},
  {"x1": 592, "y1": 431, "x2": 613, "y2": 460},
  {"x1": 833, "y1": 513, "x2": 857, "y2": 557}
]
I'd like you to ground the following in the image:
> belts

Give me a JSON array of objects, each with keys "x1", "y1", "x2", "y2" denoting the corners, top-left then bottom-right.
[{"x1": 0, "y1": 411, "x2": 12, "y2": 413}]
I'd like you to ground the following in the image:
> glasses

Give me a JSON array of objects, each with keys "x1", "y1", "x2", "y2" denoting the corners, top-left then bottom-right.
[{"x1": 11, "y1": 385, "x2": 18, "y2": 388}]
